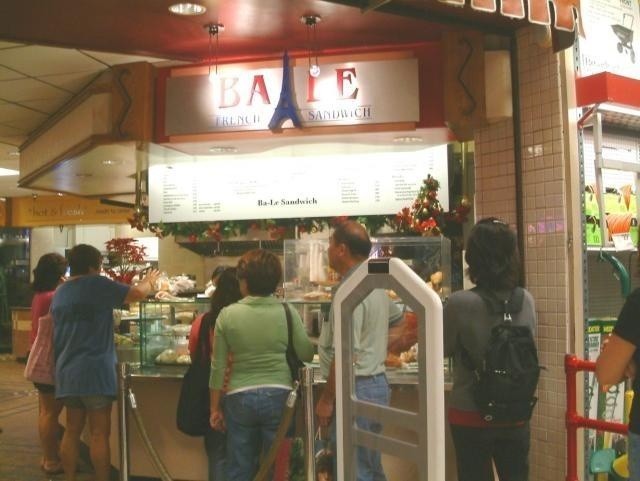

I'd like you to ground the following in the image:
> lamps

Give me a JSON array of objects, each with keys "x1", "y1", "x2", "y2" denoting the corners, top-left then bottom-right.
[{"x1": 300, "y1": 15, "x2": 321, "y2": 78}]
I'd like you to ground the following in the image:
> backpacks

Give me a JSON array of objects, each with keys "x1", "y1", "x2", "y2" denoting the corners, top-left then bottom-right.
[{"x1": 462, "y1": 287, "x2": 540, "y2": 422}]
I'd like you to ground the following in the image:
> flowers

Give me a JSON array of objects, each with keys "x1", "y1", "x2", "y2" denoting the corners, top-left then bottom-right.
[{"x1": 102, "y1": 238, "x2": 147, "y2": 275}]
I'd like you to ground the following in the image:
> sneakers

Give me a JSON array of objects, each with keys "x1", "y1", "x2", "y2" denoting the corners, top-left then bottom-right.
[{"x1": 40, "y1": 456, "x2": 80, "y2": 473}]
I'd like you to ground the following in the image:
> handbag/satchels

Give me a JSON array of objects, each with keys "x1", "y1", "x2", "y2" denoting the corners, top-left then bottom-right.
[
  {"x1": 177, "y1": 307, "x2": 211, "y2": 437},
  {"x1": 286, "y1": 346, "x2": 305, "y2": 398},
  {"x1": 24, "y1": 300, "x2": 54, "y2": 385}
]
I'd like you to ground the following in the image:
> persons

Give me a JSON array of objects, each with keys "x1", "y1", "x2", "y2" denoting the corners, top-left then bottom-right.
[
  {"x1": 313, "y1": 219, "x2": 409, "y2": 480},
  {"x1": 50, "y1": 245, "x2": 161, "y2": 480},
  {"x1": 188, "y1": 248, "x2": 314, "y2": 480},
  {"x1": 26, "y1": 252, "x2": 80, "y2": 473},
  {"x1": 595, "y1": 226, "x2": 640, "y2": 480},
  {"x1": 443, "y1": 217, "x2": 537, "y2": 481}
]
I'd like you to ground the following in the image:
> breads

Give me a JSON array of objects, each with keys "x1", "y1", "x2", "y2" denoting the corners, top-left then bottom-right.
[
  {"x1": 155, "y1": 312, "x2": 193, "y2": 364},
  {"x1": 303, "y1": 291, "x2": 331, "y2": 300}
]
